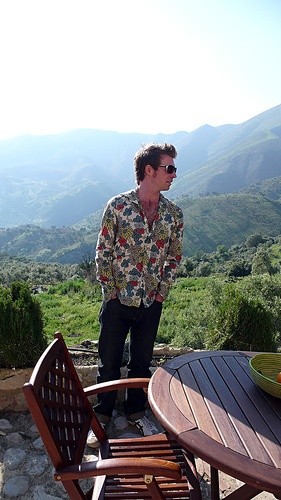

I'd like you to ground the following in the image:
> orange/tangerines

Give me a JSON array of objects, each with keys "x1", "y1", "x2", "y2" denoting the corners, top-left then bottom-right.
[{"x1": 277, "y1": 372, "x2": 281, "y2": 382}]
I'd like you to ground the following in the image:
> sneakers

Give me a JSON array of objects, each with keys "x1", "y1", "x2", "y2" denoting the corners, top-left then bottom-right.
[
  {"x1": 126, "y1": 418, "x2": 159, "y2": 439},
  {"x1": 83, "y1": 413, "x2": 109, "y2": 446}
]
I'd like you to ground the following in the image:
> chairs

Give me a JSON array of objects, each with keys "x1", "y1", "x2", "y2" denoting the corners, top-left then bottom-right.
[{"x1": 23, "y1": 331, "x2": 202, "y2": 500}]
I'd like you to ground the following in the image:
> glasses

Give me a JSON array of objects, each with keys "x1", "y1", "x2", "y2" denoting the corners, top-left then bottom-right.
[{"x1": 151, "y1": 163, "x2": 180, "y2": 175}]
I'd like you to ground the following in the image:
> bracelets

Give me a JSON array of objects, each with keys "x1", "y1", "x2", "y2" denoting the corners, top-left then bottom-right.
[{"x1": 154, "y1": 294, "x2": 163, "y2": 303}]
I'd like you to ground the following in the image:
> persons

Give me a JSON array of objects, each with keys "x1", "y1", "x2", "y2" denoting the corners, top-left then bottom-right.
[{"x1": 86, "y1": 143, "x2": 183, "y2": 448}]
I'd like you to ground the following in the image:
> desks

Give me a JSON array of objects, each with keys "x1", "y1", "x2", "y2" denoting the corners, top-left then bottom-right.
[{"x1": 147, "y1": 350, "x2": 281, "y2": 500}]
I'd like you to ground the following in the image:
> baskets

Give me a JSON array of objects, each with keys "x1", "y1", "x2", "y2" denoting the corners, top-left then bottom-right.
[{"x1": 249, "y1": 348, "x2": 281, "y2": 400}]
